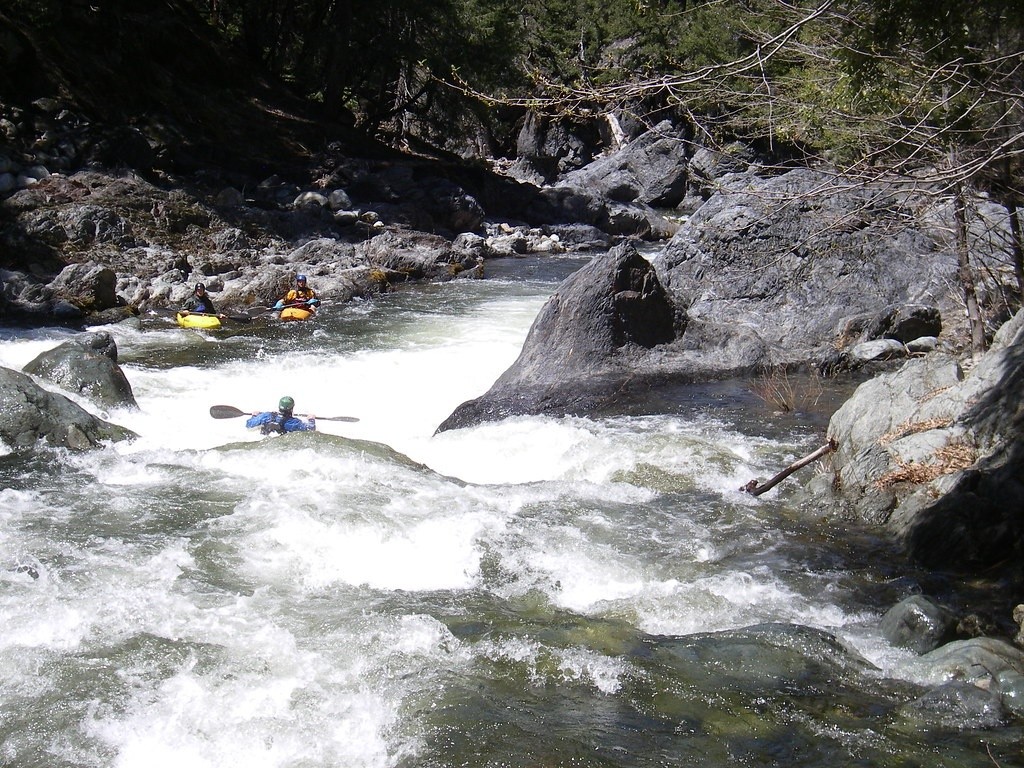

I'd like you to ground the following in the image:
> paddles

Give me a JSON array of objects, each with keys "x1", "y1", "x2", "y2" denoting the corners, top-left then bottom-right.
[
  {"x1": 247, "y1": 299, "x2": 320, "y2": 315},
  {"x1": 145, "y1": 308, "x2": 251, "y2": 322},
  {"x1": 209, "y1": 405, "x2": 360, "y2": 423}
]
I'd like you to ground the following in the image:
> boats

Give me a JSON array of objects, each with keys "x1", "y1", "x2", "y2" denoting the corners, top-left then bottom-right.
[
  {"x1": 281, "y1": 307, "x2": 312, "y2": 322},
  {"x1": 176, "y1": 312, "x2": 222, "y2": 330}
]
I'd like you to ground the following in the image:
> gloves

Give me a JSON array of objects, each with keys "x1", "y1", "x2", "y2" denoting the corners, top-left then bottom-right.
[
  {"x1": 276, "y1": 302, "x2": 284, "y2": 310},
  {"x1": 308, "y1": 299, "x2": 318, "y2": 305}
]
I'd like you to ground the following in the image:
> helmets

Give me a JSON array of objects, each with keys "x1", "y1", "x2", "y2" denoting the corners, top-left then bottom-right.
[
  {"x1": 279, "y1": 396, "x2": 294, "y2": 410},
  {"x1": 195, "y1": 283, "x2": 204, "y2": 291},
  {"x1": 297, "y1": 275, "x2": 306, "y2": 281}
]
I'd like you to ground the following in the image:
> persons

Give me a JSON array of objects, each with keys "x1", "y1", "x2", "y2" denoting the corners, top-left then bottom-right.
[
  {"x1": 246, "y1": 397, "x2": 316, "y2": 433},
  {"x1": 274, "y1": 276, "x2": 321, "y2": 314},
  {"x1": 179, "y1": 283, "x2": 228, "y2": 319}
]
